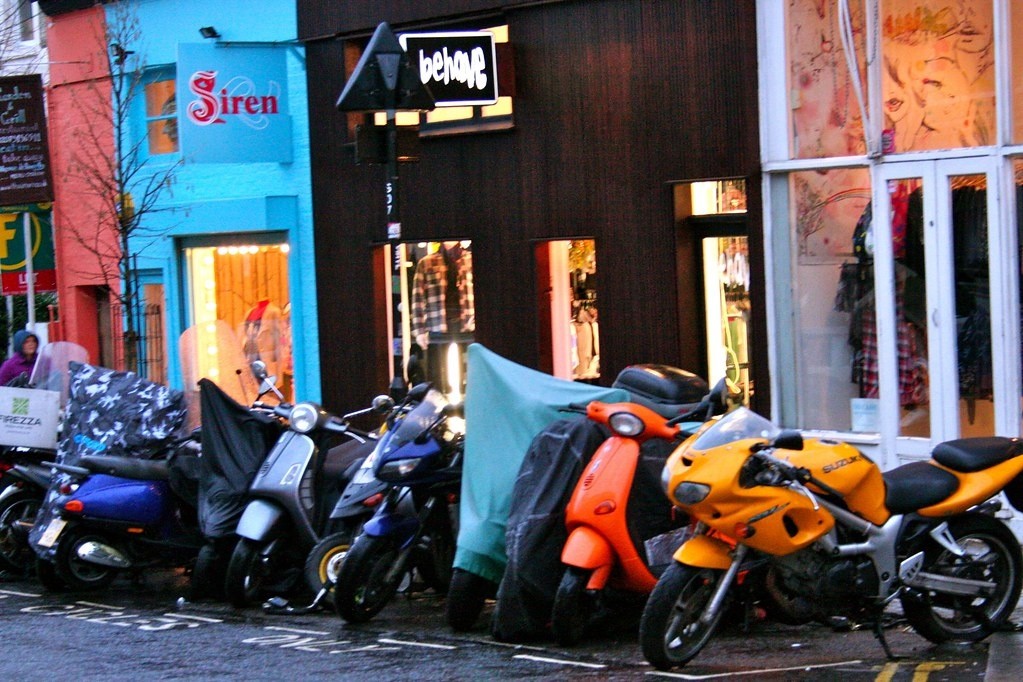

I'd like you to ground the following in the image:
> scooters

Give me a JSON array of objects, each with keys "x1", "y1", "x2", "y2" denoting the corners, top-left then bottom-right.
[
  {"x1": 0, "y1": 341, "x2": 712, "y2": 647},
  {"x1": 550, "y1": 379, "x2": 817, "y2": 648}
]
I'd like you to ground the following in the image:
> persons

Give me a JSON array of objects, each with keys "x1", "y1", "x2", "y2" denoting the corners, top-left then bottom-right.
[
  {"x1": 236, "y1": 297, "x2": 283, "y2": 399},
  {"x1": 411, "y1": 242, "x2": 475, "y2": 398},
  {"x1": 0, "y1": 330, "x2": 52, "y2": 386}
]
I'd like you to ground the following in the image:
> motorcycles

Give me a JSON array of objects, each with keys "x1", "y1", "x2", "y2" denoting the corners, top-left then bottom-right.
[{"x1": 637, "y1": 403, "x2": 1023, "y2": 671}]
